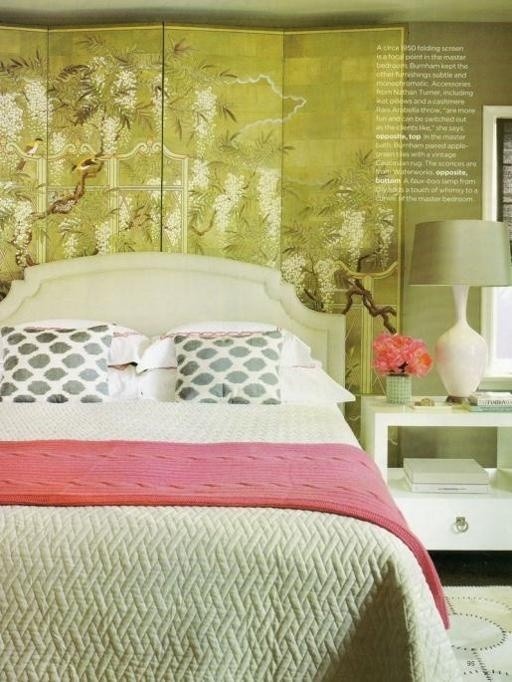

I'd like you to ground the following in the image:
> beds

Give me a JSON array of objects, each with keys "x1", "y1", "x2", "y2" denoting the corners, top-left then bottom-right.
[{"x1": 1, "y1": 251, "x2": 432, "y2": 682}]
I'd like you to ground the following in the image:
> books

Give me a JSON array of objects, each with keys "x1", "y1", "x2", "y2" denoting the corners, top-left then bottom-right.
[
  {"x1": 462, "y1": 399, "x2": 511, "y2": 413},
  {"x1": 404, "y1": 473, "x2": 490, "y2": 494},
  {"x1": 470, "y1": 392, "x2": 512, "y2": 405},
  {"x1": 403, "y1": 457, "x2": 489, "y2": 483}
]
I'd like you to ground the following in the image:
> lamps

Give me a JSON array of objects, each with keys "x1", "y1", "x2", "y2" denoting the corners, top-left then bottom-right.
[{"x1": 408, "y1": 220, "x2": 511, "y2": 400}]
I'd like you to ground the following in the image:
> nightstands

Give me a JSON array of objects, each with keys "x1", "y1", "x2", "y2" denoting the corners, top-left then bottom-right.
[{"x1": 360, "y1": 391, "x2": 512, "y2": 554}]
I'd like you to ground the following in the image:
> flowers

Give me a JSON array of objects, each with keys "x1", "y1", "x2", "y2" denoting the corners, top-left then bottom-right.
[{"x1": 374, "y1": 335, "x2": 430, "y2": 376}]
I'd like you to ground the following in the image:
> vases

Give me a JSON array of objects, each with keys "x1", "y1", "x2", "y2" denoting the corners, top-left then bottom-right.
[{"x1": 385, "y1": 375, "x2": 412, "y2": 405}]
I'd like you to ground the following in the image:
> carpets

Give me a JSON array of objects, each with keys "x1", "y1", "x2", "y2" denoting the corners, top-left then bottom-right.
[{"x1": 432, "y1": 584, "x2": 512, "y2": 677}]
